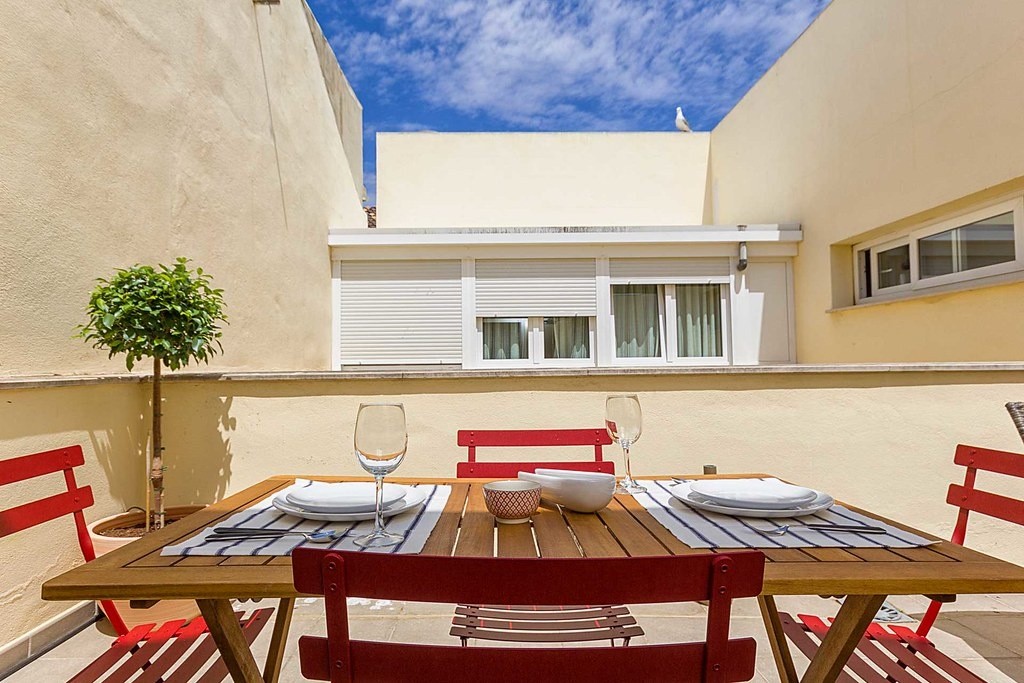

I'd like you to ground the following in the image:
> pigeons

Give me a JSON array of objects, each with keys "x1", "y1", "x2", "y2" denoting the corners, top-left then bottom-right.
[{"x1": 674, "y1": 106, "x2": 693, "y2": 133}]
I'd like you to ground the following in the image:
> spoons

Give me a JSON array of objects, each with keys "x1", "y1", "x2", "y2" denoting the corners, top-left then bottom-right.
[{"x1": 204, "y1": 528, "x2": 332, "y2": 543}]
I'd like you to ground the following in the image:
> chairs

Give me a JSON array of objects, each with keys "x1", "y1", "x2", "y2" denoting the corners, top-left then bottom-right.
[
  {"x1": 292, "y1": 547, "x2": 765, "y2": 683},
  {"x1": 447, "y1": 429, "x2": 645, "y2": 647}
]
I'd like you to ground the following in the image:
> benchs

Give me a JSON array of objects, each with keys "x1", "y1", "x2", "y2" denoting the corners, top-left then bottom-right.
[
  {"x1": 0, "y1": 445, "x2": 274, "y2": 683},
  {"x1": 776, "y1": 444, "x2": 1024, "y2": 683}
]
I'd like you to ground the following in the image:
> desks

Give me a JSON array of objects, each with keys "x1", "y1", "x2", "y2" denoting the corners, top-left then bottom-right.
[{"x1": 41, "y1": 475, "x2": 1024, "y2": 683}]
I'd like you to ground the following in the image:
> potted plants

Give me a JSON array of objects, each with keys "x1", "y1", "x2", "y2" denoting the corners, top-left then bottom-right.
[{"x1": 70, "y1": 257, "x2": 231, "y2": 633}]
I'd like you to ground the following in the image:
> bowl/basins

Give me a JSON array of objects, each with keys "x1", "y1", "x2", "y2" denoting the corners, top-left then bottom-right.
[{"x1": 482, "y1": 481, "x2": 542, "y2": 524}]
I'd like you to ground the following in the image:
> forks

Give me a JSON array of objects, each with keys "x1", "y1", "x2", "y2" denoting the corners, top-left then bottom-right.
[
  {"x1": 214, "y1": 528, "x2": 348, "y2": 537},
  {"x1": 744, "y1": 521, "x2": 886, "y2": 537}
]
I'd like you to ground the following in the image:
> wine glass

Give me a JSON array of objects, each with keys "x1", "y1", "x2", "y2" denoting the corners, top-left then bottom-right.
[
  {"x1": 605, "y1": 394, "x2": 647, "y2": 494},
  {"x1": 354, "y1": 402, "x2": 408, "y2": 547}
]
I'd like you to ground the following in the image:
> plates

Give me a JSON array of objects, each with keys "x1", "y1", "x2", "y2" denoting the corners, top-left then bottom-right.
[
  {"x1": 272, "y1": 486, "x2": 426, "y2": 521},
  {"x1": 671, "y1": 482, "x2": 834, "y2": 517},
  {"x1": 690, "y1": 480, "x2": 817, "y2": 510},
  {"x1": 286, "y1": 482, "x2": 407, "y2": 512}
]
